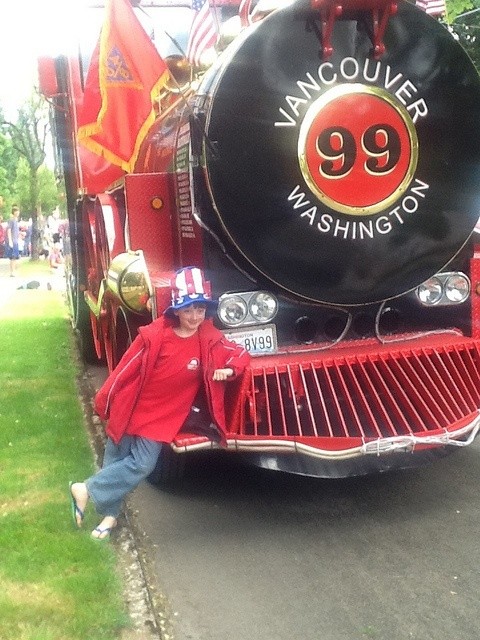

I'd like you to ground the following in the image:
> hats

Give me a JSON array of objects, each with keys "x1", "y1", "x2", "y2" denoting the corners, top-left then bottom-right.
[{"x1": 163, "y1": 266, "x2": 218, "y2": 319}]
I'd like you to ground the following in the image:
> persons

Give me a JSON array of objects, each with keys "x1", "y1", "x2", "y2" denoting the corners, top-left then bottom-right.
[
  {"x1": 4, "y1": 206, "x2": 21, "y2": 278},
  {"x1": 67, "y1": 265, "x2": 253, "y2": 542}
]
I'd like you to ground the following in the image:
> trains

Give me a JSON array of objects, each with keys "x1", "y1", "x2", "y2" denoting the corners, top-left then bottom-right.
[{"x1": 38, "y1": 0, "x2": 480, "y2": 486}]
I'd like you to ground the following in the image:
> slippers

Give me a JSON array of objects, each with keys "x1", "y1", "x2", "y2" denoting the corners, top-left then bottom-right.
[
  {"x1": 69, "y1": 481, "x2": 84, "y2": 529},
  {"x1": 90, "y1": 521, "x2": 119, "y2": 543}
]
